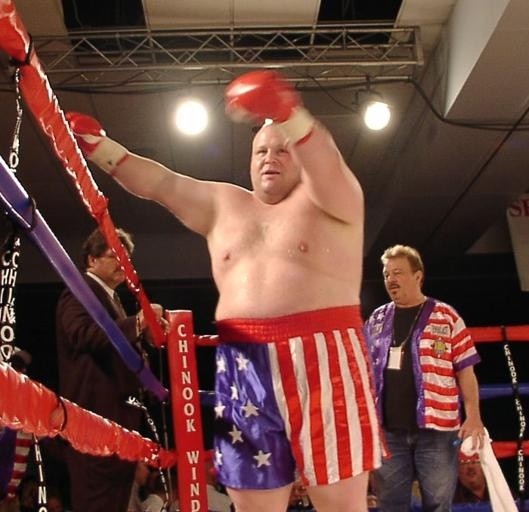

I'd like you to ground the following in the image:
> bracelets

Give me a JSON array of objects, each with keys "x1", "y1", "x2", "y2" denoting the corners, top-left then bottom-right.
[{"x1": 136, "y1": 314, "x2": 141, "y2": 334}]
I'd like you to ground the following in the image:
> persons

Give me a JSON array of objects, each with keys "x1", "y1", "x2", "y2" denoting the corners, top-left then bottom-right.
[
  {"x1": 53, "y1": 223, "x2": 169, "y2": 512},
  {"x1": 64, "y1": 68, "x2": 392, "y2": 512},
  {"x1": 362, "y1": 242, "x2": 487, "y2": 512},
  {"x1": 0, "y1": 344, "x2": 521, "y2": 512}
]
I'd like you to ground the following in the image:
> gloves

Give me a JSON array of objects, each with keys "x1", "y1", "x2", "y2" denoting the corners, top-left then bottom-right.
[
  {"x1": 41, "y1": 107, "x2": 131, "y2": 180},
  {"x1": 222, "y1": 69, "x2": 319, "y2": 149}
]
